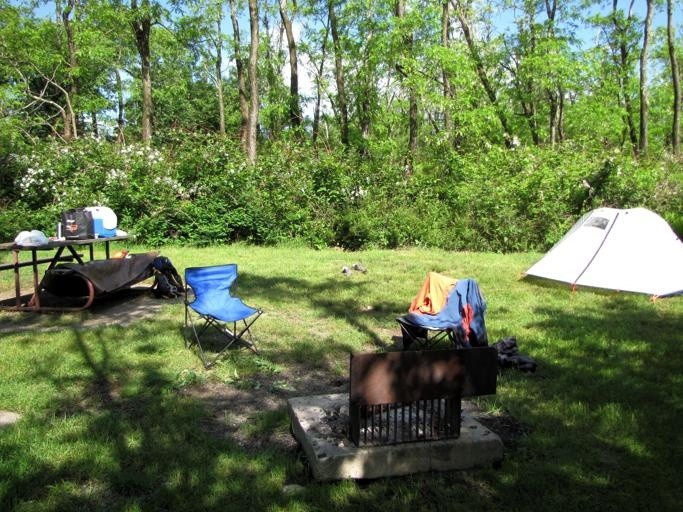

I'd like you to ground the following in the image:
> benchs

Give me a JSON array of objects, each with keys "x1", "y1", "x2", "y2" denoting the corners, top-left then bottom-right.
[{"x1": 0, "y1": 253, "x2": 84, "y2": 270}]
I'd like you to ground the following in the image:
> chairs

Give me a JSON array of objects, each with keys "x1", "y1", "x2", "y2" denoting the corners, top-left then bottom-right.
[
  {"x1": 396, "y1": 272, "x2": 475, "y2": 349},
  {"x1": 185, "y1": 264, "x2": 264, "y2": 370}
]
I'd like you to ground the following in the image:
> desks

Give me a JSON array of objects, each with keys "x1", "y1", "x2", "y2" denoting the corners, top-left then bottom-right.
[{"x1": 0, "y1": 229, "x2": 129, "y2": 313}]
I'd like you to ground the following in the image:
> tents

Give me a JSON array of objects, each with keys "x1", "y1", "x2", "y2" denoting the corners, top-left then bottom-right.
[{"x1": 520, "y1": 205, "x2": 683, "y2": 307}]
